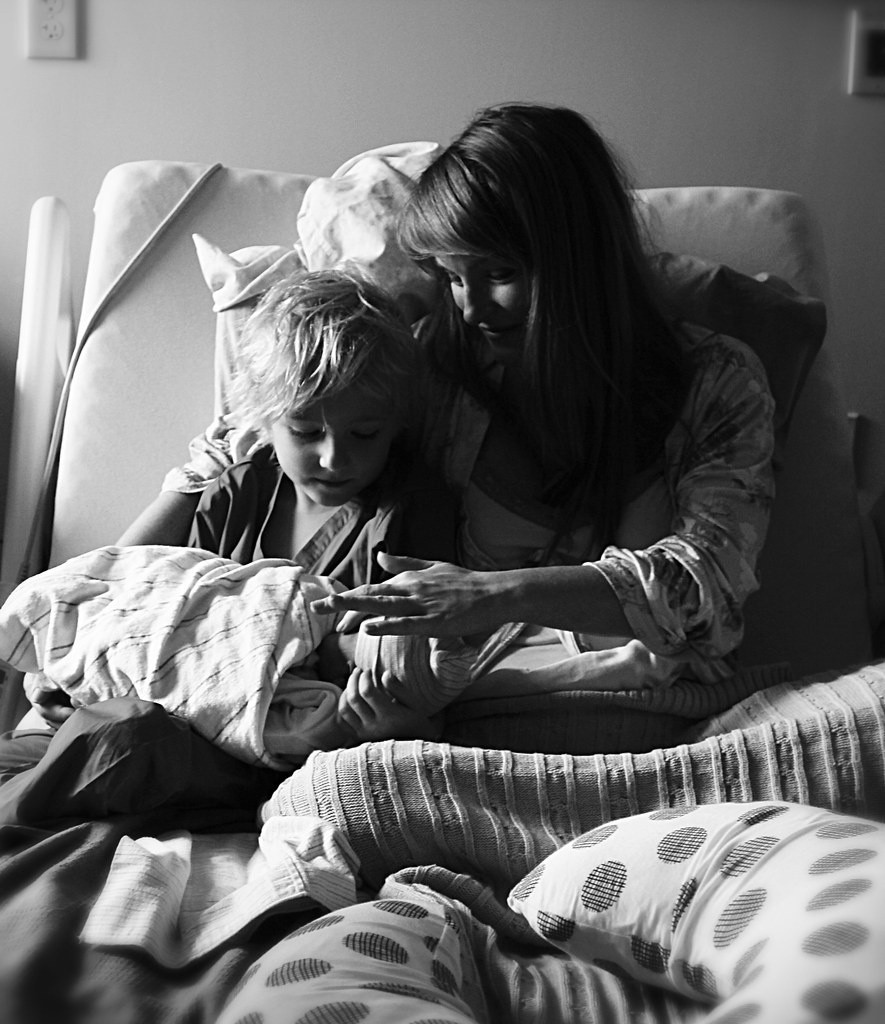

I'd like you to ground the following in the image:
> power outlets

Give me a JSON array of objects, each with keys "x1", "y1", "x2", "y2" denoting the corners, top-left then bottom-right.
[{"x1": 26, "y1": 0, "x2": 80, "y2": 60}]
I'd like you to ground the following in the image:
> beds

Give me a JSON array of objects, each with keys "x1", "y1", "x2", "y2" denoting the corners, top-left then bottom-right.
[{"x1": 0, "y1": 158, "x2": 884, "y2": 1024}]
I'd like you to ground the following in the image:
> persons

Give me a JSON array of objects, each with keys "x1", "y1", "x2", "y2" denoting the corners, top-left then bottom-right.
[{"x1": 0, "y1": 102, "x2": 885, "y2": 898}]
